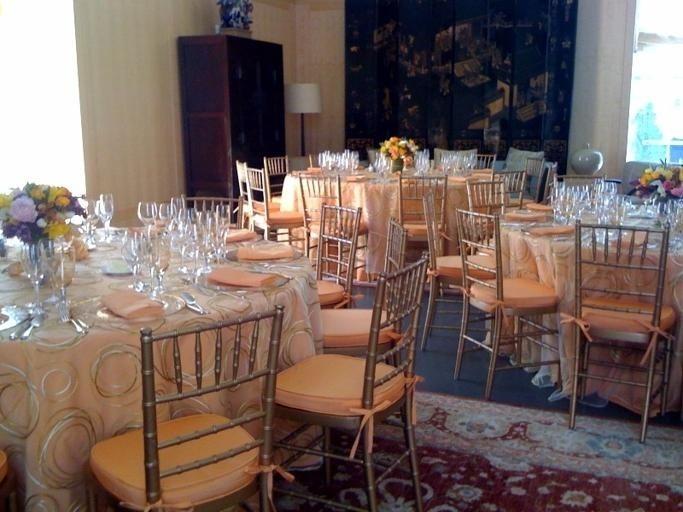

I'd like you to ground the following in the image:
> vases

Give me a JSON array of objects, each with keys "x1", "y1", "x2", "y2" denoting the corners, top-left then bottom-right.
[
  {"x1": 220, "y1": 29, "x2": 250, "y2": 37},
  {"x1": 25, "y1": 240, "x2": 53, "y2": 286}
]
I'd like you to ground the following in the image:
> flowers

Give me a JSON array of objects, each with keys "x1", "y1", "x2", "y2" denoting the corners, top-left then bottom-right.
[
  {"x1": 0, "y1": 182, "x2": 86, "y2": 243},
  {"x1": 622, "y1": 158, "x2": 682, "y2": 206},
  {"x1": 216, "y1": 1, "x2": 254, "y2": 28}
]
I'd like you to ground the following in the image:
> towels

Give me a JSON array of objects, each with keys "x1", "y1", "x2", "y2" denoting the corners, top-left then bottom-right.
[
  {"x1": 208, "y1": 265, "x2": 274, "y2": 288},
  {"x1": 225, "y1": 243, "x2": 295, "y2": 261},
  {"x1": 100, "y1": 288, "x2": 163, "y2": 322}
]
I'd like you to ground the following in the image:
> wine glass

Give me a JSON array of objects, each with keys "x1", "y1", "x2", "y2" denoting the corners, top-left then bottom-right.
[
  {"x1": 0, "y1": 188, "x2": 308, "y2": 341},
  {"x1": 316, "y1": 149, "x2": 478, "y2": 176},
  {"x1": 501, "y1": 180, "x2": 683, "y2": 245}
]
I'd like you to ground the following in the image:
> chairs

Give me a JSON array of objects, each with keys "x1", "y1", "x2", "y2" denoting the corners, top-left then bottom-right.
[
  {"x1": 319, "y1": 217, "x2": 409, "y2": 378},
  {"x1": 272, "y1": 253, "x2": 433, "y2": 512},
  {"x1": 566, "y1": 216, "x2": 680, "y2": 442},
  {"x1": 88, "y1": 303, "x2": 284, "y2": 512},
  {"x1": 421, "y1": 193, "x2": 499, "y2": 356},
  {"x1": 448, "y1": 205, "x2": 567, "y2": 404},
  {"x1": 232, "y1": 134, "x2": 558, "y2": 310}
]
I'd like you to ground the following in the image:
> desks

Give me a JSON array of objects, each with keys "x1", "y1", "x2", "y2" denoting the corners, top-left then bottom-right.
[{"x1": 487, "y1": 197, "x2": 680, "y2": 425}]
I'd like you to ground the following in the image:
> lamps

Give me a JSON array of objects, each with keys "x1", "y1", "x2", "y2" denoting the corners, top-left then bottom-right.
[{"x1": 285, "y1": 81, "x2": 323, "y2": 157}]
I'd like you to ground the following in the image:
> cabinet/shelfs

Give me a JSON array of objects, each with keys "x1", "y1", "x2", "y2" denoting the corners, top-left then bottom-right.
[{"x1": 176, "y1": 36, "x2": 286, "y2": 221}]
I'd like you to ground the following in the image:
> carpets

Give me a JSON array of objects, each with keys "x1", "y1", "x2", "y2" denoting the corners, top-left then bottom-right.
[{"x1": 272, "y1": 388, "x2": 681, "y2": 512}]
